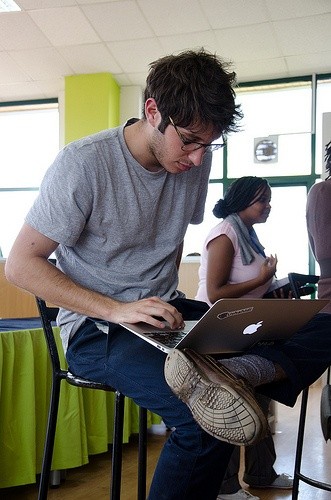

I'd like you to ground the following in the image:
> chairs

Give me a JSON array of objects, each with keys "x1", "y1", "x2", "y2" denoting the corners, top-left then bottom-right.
[{"x1": 36, "y1": 259, "x2": 149, "y2": 499}]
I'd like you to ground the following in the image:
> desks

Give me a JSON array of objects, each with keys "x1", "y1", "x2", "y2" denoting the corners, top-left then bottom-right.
[{"x1": 0, "y1": 314, "x2": 163, "y2": 488}]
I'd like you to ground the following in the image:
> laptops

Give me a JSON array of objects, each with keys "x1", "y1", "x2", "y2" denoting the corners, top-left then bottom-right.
[{"x1": 117, "y1": 298, "x2": 330, "y2": 355}]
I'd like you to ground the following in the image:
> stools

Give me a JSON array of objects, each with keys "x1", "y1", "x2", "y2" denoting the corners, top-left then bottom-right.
[{"x1": 287, "y1": 272, "x2": 331, "y2": 500}]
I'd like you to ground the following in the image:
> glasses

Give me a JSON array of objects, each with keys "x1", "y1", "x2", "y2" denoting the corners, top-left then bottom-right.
[{"x1": 168, "y1": 116, "x2": 226, "y2": 152}]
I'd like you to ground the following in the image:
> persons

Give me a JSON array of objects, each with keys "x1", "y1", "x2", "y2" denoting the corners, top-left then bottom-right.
[
  {"x1": 306, "y1": 140, "x2": 331, "y2": 300},
  {"x1": 196, "y1": 176, "x2": 293, "y2": 500},
  {"x1": 5, "y1": 51, "x2": 266, "y2": 500}
]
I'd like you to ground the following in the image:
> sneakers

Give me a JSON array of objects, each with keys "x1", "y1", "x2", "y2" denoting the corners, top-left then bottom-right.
[
  {"x1": 217, "y1": 489, "x2": 260, "y2": 500},
  {"x1": 164, "y1": 348, "x2": 268, "y2": 446},
  {"x1": 251, "y1": 473, "x2": 293, "y2": 488}
]
